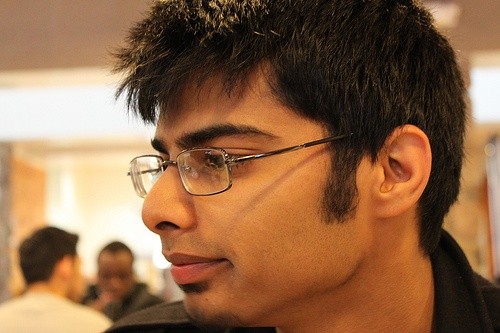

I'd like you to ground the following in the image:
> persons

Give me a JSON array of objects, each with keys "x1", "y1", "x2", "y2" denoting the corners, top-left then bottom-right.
[
  {"x1": 0, "y1": 226, "x2": 113, "y2": 332},
  {"x1": 101, "y1": 0, "x2": 500, "y2": 333},
  {"x1": 82, "y1": 242, "x2": 164, "y2": 322}
]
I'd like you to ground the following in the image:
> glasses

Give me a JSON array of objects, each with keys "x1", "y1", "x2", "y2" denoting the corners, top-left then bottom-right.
[{"x1": 127, "y1": 132, "x2": 360, "y2": 196}]
